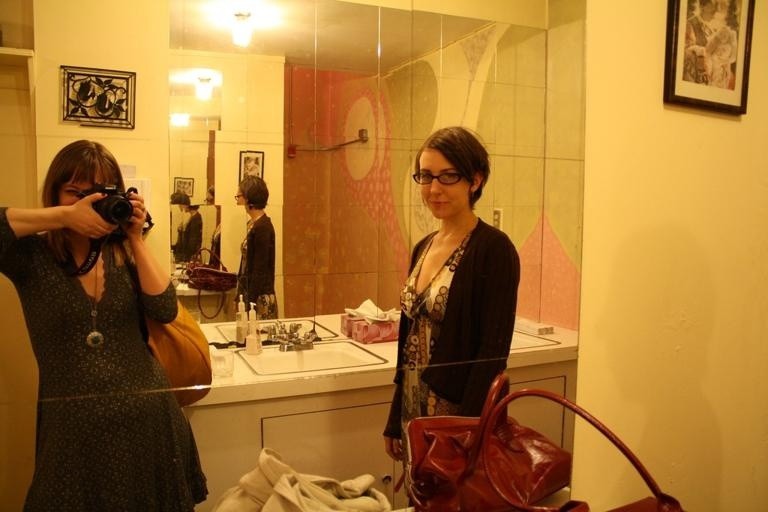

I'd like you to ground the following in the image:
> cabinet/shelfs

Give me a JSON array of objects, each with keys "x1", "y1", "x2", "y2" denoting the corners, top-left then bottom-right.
[{"x1": 260, "y1": 362, "x2": 579, "y2": 511}]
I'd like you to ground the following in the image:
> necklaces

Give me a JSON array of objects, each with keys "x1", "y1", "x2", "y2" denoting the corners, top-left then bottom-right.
[{"x1": 77, "y1": 261, "x2": 105, "y2": 348}]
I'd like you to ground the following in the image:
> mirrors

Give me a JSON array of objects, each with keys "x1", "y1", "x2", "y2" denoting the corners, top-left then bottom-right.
[
  {"x1": 35, "y1": 0, "x2": 586, "y2": 351},
  {"x1": 170, "y1": 202, "x2": 221, "y2": 269}
]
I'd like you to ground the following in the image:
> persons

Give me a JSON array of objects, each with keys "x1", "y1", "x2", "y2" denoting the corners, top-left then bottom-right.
[
  {"x1": 382, "y1": 128, "x2": 520, "y2": 506},
  {"x1": 0, "y1": 140, "x2": 210, "y2": 512},
  {"x1": 205, "y1": 185, "x2": 215, "y2": 205},
  {"x1": 234, "y1": 175, "x2": 279, "y2": 321},
  {"x1": 209, "y1": 223, "x2": 220, "y2": 268},
  {"x1": 171, "y1": 192, "x2": 190, "y2": 206},
  {"x1": 170, "y1": 205, "x2": 202, "y2": 263}
]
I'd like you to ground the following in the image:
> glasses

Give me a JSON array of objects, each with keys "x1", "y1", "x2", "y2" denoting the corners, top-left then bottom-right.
[
  {"x1": 233, "y1": 194, "x2": 245, "y2": 201},
  {"x1": 412, "y1": 169, "x2": 466, "y2": 184}
]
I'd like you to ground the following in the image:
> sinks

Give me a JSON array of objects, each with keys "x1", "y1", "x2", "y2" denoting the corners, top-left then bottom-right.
[
  {"x1": 236, "y1": 341, "x2": 387, "y2": 378},
  {"x1": 214, "y1": 318, "x2": 339, "y2": 343}
]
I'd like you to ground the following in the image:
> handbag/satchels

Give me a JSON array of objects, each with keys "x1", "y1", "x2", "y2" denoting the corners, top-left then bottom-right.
[
  {"x1": 480, "y1": 387, "x2": 688, "y2": 511},
  {"x1": 398, "y1": 371, "x2": 573, "y2": 511},
  {"x1": 143, "y1": 278, "x2": 214, "y2": 409},
  {"x1": 188, "y1": 247, "x2": 239, "y2": 320}
]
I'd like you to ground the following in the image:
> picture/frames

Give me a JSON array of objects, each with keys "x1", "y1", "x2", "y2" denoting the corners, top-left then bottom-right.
[
  {"x1": 173, "y1": 176, "x2": 194, "y2": 198},
  {"x1": 663, "y1": 1, "x2": 755, "y2": 115},
  {"x1": 61, "y1": 64, "x2": 135, "y2": 130},
  {"x1": 239, "y1": 151, "x2": 264, "y2": 182}
]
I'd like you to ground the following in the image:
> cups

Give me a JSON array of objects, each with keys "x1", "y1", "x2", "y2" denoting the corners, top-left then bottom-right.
[
  {"x1": 189, "y1": 309, "x2": 200, "y2": 325},
  {"x1": 214, "y1": 354, "x2": 235, "y2": 380}
]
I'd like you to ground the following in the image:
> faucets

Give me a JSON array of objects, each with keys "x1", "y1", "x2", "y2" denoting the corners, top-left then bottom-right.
[
  {"x1": 256, "y1": 320, "x2": 287, "y2": 342},
  {"x1": 278, "y1": 322, "x2": 321, "y2": 352}
]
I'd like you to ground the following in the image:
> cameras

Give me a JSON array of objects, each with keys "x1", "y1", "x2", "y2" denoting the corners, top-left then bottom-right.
[{"x1": 82, "y1": 185, "x2": 133, "y2": 228}]
